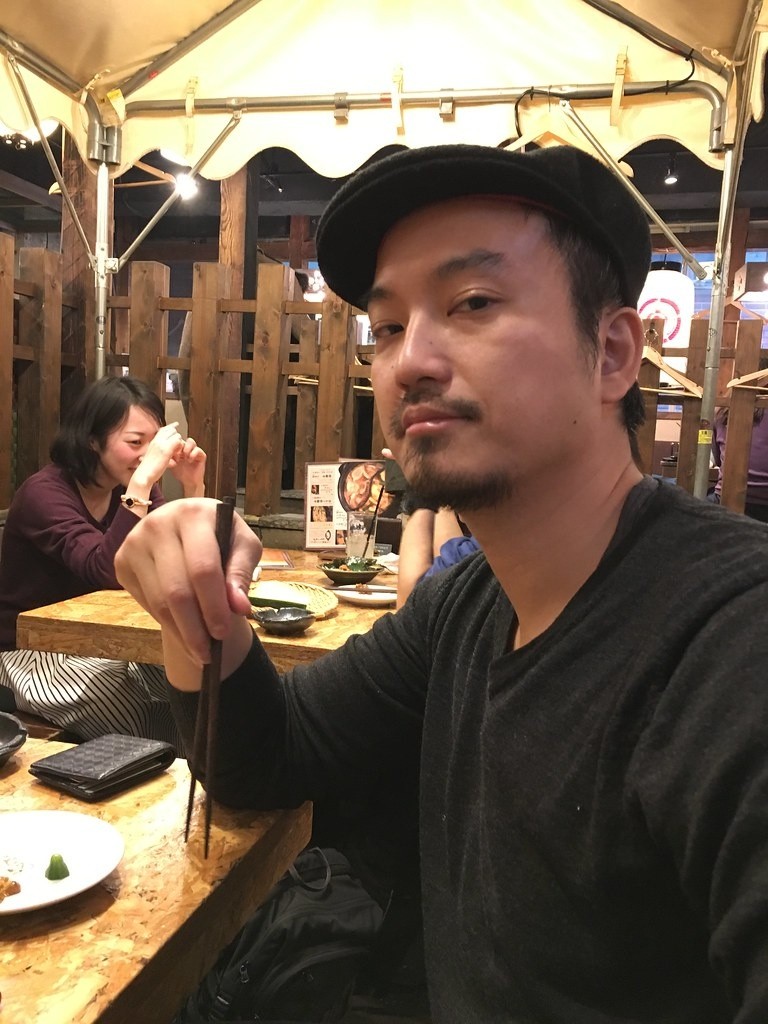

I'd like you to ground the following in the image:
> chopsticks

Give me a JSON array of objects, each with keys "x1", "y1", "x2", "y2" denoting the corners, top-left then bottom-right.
[
  {"x1": 323, "y1": 587, "x2": 398, "y2": 594},
  {"x1": 185, "y1": 496, "x2": 246, "y2": 860}
]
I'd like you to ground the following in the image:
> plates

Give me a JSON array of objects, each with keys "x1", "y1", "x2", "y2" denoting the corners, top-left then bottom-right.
[
  {"x1": 333, "y1": 584, "x2": 398, "y2": 607},
  {"x1": 0, "y1": 810, "x2": 125, "y2": 917}
]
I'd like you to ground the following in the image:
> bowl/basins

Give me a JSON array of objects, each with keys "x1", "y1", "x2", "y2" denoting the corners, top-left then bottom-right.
[
  {"x1": 316, "y1": 563, "x2": 384, "y2": 586},
  {"x1": 337, "y1": 461, "x2": 400, "y2": 519}
]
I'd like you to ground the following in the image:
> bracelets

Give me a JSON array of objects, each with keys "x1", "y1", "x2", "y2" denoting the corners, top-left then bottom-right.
[{"x1": 121, "y1": 495, "x2": 153, "y2": 508}]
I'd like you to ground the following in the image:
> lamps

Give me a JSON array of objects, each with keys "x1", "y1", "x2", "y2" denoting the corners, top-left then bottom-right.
[{"x1": 662, "y1": 152, "x2": 679, "y2": 186}]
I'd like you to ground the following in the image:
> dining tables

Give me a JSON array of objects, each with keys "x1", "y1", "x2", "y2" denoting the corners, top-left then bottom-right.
[
  {"x1": 0, "y1": 739, "x2": 313, "y2": 1024},
  {"x1": 16, "y1": 546, "x2": 398, "y2": 678}
]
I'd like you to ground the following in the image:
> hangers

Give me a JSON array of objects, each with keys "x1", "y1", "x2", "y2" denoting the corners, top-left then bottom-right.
[
  {"x1": 727, "y1": 369, "x2": 768, "y2": 391},
  {"x1": 691, "y1": 295, "x2": 768, "y2": 325},
  {"x1": 636, "y1": 328, "x2": 704, "y2": 398}
]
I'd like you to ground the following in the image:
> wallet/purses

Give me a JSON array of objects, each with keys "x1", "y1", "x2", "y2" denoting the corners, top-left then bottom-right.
[{"x1": 28, "y1": 733, "x2": 177, "y2": 803}]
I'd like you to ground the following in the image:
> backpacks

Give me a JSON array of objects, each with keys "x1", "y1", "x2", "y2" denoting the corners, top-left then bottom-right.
[{"x1": 175, "y1": 846, "x2": 432, "y2": 1024}]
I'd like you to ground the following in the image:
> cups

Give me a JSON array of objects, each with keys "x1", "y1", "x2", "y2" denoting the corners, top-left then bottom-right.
[{"x1": 346, "y1": 510, "x2": 377, "y2": 560}]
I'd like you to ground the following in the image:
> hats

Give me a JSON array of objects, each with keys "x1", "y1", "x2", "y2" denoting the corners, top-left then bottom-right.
[{"x1": 314, "y1": 144, "x2": 653, "y2": 313}]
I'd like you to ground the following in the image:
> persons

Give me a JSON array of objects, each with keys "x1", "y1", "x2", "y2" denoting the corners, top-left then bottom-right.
[
  {"x1": 114, "y1": 145, "x2": 768, "y2": 1024},
  {"x1": 0, "y1": 375, "x2": 206, "y2": 715}
]
